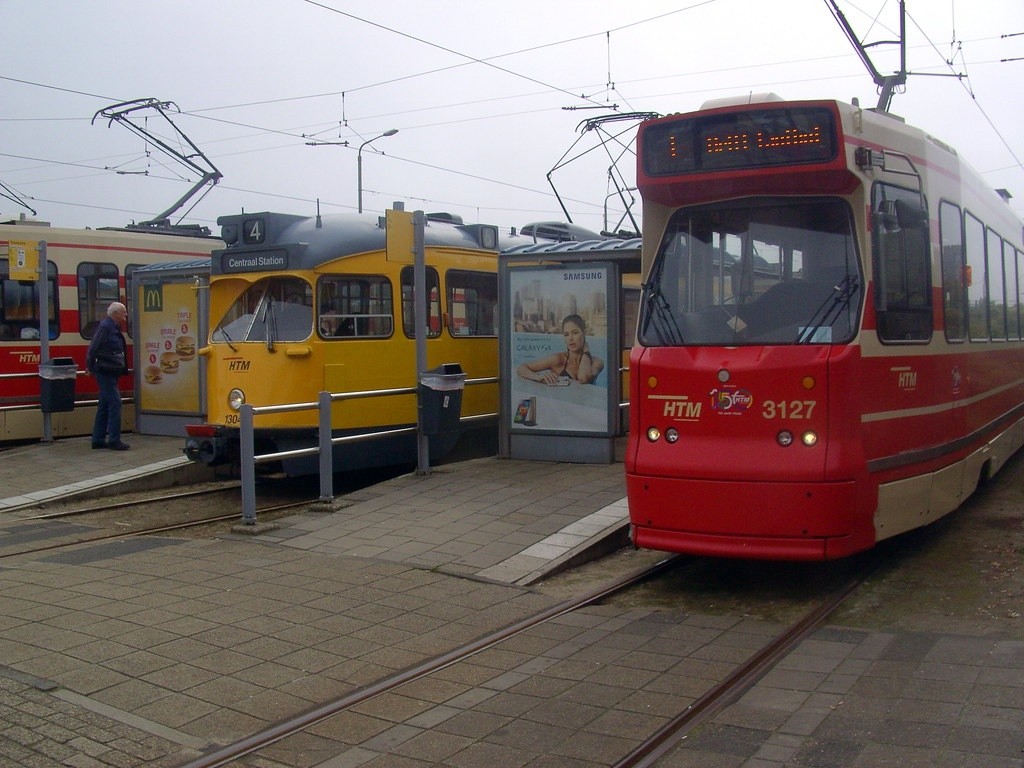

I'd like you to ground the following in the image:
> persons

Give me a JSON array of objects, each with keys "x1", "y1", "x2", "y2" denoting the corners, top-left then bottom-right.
[
  {"x1": 335, "y1": 301, "x2": 361, "y2": 336},
  {"x1": 515, "y1": 314, "x2": 605, "y2": 385},
  {"x1": 85, "y1": 302, "x2": 130, "y2": 450}
]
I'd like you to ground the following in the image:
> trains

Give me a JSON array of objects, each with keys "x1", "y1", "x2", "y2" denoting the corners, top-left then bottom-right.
[
  {"x1": 180, "y1": 202, "x2": 802, "y2": 480},
  {"x1": 0, "y1": 217, "x2": 463, "y2": 442},
  {"x1": 621, "y1": 93, "x2": 1022, "y2": 560}
]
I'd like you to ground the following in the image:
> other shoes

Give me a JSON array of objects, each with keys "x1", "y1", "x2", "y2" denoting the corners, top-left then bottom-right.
[
  {"x1": 107, "y1": 440, "x2": 130, "y2": 450},
  {"x1": 91, "y1": 440, "x2": 108, "y2": 449}
]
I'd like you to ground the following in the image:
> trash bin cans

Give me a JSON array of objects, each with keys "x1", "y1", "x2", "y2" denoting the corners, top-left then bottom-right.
[
  {"x1": 418, "y1": 362, "x2": 468, "y2": 436},
  {"x1": 39, "y1": 357, "x2": 79, "y2": 412}
]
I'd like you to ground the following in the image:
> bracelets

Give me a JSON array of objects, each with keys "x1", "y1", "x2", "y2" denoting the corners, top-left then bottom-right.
[{"x1": 582, "y1": 351, "x2": 591, "y2": 358}]
